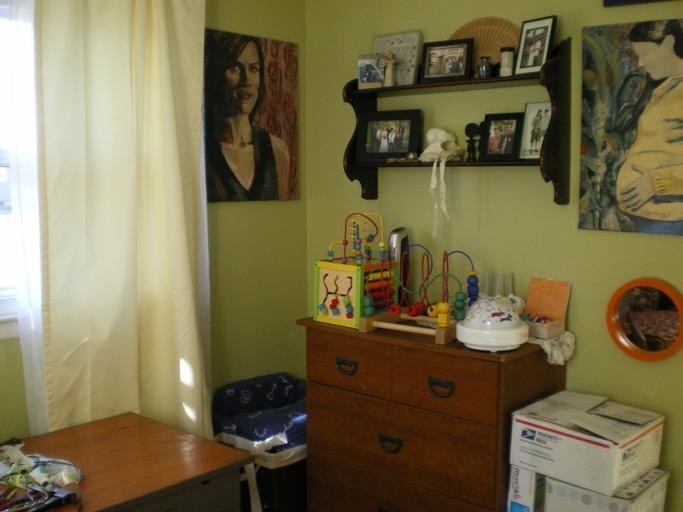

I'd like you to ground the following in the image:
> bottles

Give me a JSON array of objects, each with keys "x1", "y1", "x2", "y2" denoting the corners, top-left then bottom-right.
[
  {"x1": 498, "y1": 47, "x2": 514, "y2": 76},
  {"x1": 477, "y1": 57, "x2": 492, "y2": 80}
]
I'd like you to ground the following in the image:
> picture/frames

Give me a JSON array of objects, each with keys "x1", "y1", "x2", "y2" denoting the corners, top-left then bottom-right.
[
  {"x1": 357, "y1": 106, "x2": 428, "y2": 163},
  {"x1": 477, "y1": 108, "x2": 523, "y2": 159},
  {"x1": 509, "y1": 15, "x2": 558, "y2": 75},
  {"x1": 416, "y1": 33, "x2": 475, "y2": 81},
  {"x1": 517, "y1": 100, "x2": 552, "y2": 162}
]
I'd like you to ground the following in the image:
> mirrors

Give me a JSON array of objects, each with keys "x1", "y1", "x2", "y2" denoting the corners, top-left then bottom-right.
[{"x1": 605, "y1": 277, "x2": 682, "y2": 360}]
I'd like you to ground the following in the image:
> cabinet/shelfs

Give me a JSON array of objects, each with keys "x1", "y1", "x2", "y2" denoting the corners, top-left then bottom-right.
[
  {"x1": 291, "y1": 305, "x2": 568, "y2": 512},
  {"x1": 342, "y1": 33, "x2": 572, "y2": 206},
  {"x1": 0, "y1": 409, "x2": 259, "y2": 512}
]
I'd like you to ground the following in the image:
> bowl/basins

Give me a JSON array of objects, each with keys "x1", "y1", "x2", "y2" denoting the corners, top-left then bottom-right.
[{"x1": 455, "y1": 320, "x2": 530, "y2": 352}]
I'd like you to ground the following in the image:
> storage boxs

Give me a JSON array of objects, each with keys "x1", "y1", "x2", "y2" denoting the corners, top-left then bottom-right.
[
  {"x1": 506, "y1": 386, "x2": 667, "y2": 495},
  {"x1": 505, "y1": 459, "x2": 671, "y2": 511}
]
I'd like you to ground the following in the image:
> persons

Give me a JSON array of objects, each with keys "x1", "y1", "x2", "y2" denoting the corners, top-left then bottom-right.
[
  {"x1": 434, "y1": 52, "x2": 461, "y2": 74},
  {"x1": 526, "y1": 39, "x2": 535, "y2": 67},
  {"x1": 538, "y1": 108, "x2": 550, "y2": 137},
  {"x1": 203, "y1": 28, "x2": 291, "y2": 202},
  {"x1": 612, "y1": 19, "x2": 682, "y2": 235},
  {"x1": 371, "y1": 122, "x2": 405, "y2": 154},
  {"x1": 376, "y1": 51, "x2": 397, "y2": 88},
  {"x1": 533, "y1": 36, "x2": 541, "y2": 65},
  {"x1": 497, "y1": 121, "x2": 514, "y2": 153},
  {"x1": 528, "y1": 108, "x2": 542, "y2": 153},
  {"x1": 487, "y1": 125, "x2": 503, "y2": 154}
]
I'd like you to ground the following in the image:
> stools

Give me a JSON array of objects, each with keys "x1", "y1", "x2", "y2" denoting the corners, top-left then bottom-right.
[{"x1": 204, "y1": 363, "x2": 310, "y2": 512}]
